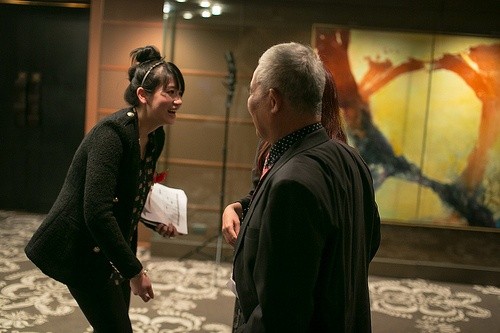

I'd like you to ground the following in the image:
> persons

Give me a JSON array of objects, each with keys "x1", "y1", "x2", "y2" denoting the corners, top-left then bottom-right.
[
  {"x1": 24, "y1": 44, "x2": 185, "y2": 333},
  {"x1": 222, "y1": 41, "x2": 381, "y2": 333}
]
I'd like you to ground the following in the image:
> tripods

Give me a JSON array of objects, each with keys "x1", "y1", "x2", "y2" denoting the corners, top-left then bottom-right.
[{"x1": 176, "y1": 71, "x2": 238, "y2": 288}]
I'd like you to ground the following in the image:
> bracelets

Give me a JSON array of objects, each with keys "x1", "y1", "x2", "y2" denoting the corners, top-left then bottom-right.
[{"x1": 129, "y1": 270, "x2": 147, "y2": 281}]
